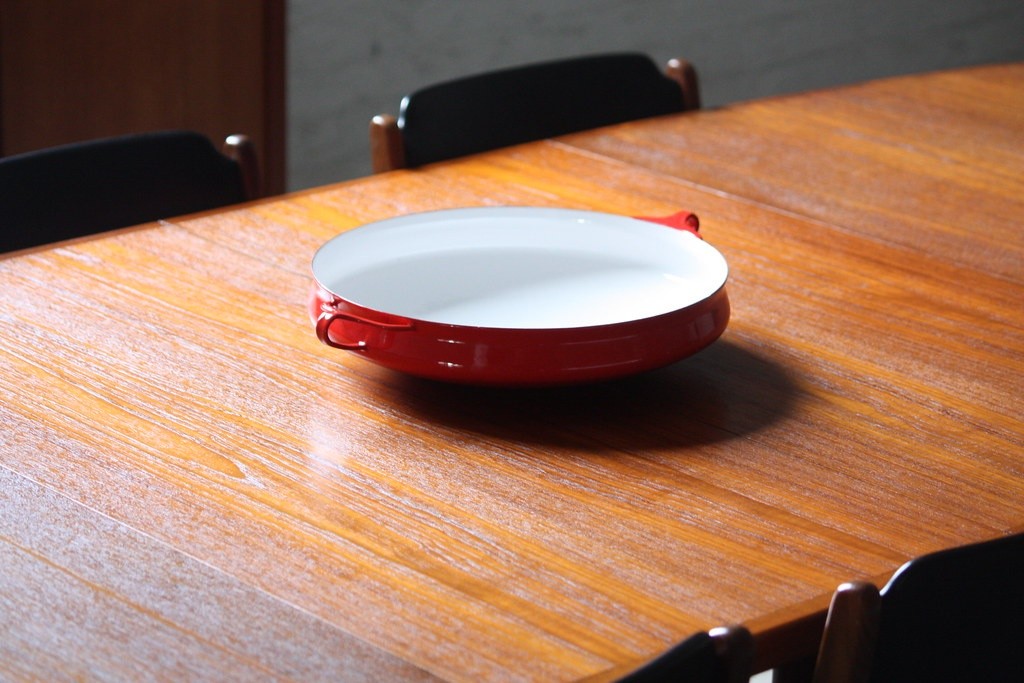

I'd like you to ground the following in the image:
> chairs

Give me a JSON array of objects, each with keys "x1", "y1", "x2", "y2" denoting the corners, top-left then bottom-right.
[
  {"x1": 367, "y1": 51, "x2": 701, "y2": 174},
  {"x1": 0, "y1": 133, "x2": 261, "y2": 256}
]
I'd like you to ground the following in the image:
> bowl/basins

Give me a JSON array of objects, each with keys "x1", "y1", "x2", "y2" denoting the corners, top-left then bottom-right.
[{"x1": 308, "y1": 206, "x2": 730, "y2": 387}]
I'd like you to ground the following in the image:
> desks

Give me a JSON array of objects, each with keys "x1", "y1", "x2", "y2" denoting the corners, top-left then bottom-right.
[{"x1": 0, "y1": 62, "x2": 1024, "y2": 683}]
